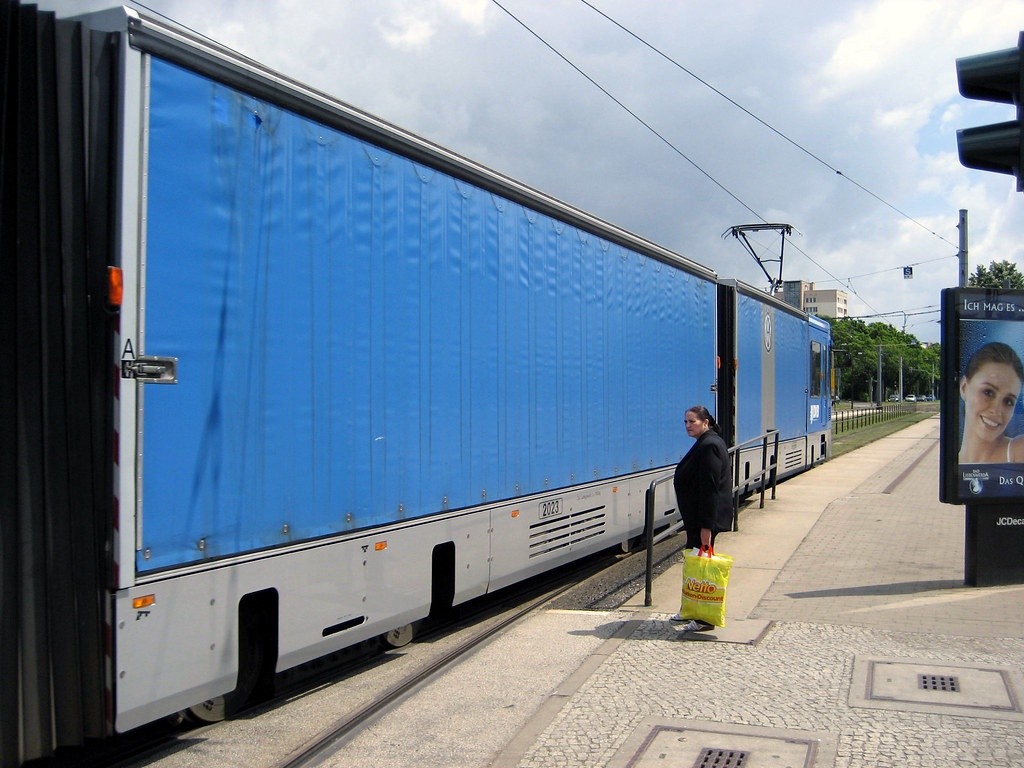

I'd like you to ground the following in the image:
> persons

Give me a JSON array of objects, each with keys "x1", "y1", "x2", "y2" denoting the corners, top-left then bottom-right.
[
  {"x1": 958, "y1": 341, "x2": 1024, "y2": 465},
  {"x1": 668, "y1": 406, "x2": 733, "y2": 632}
]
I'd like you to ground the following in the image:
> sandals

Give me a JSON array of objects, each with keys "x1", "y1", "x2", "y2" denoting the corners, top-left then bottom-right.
[
  {"x1": 680, "y1": 619, "x2": 714, "y2": 632},
  {"x1": 669, "y1": 613, "x2": 694, "y2": 621}
]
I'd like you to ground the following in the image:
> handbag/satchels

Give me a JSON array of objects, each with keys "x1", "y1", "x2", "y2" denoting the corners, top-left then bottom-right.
[{"x1": 678, "y1": 545, "x2": 734, "y2": 628}]
